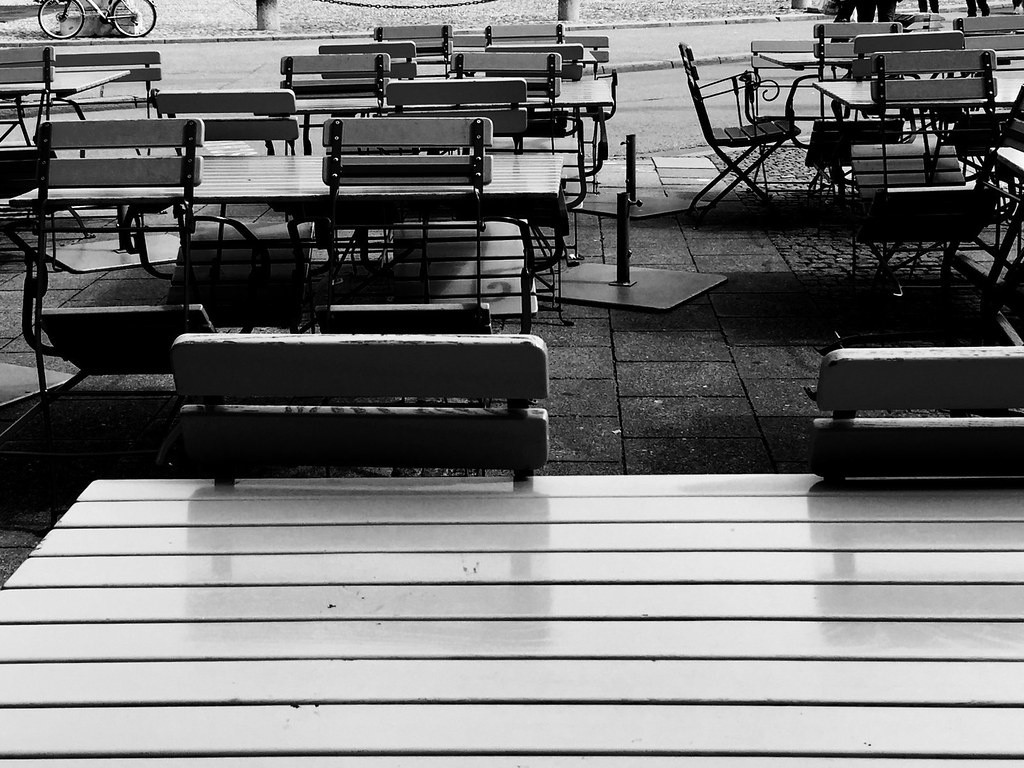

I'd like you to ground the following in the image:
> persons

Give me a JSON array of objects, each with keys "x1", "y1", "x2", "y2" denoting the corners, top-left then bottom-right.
[
  {"x1": 854, "y1": 0, "x2": 903, "y2": 22},
  {"x1": 1012, "y1": 0, "x2": 1024, "y2": 15},
  {"x1": 966, "y1": 0, "x2": 990, "y2": 17},
  {"x1": 917, "y1": 0, "x2": 940, "y2": 13}
]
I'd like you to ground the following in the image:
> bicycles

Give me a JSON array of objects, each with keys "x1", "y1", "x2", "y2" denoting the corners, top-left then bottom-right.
[{"x1": 34, "y1": 0, "x2": 157, "y2": 38}]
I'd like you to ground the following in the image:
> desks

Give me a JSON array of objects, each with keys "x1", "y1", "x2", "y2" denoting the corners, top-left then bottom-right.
[
  {"x1": 8, "y1": 157, "x2": 564, "y2": 402},
  {"x1": 0, "y1": 476, "x2": 1024, "y2": 768},
  {"x1": 0, "y1": 71, "x2": 131, "y2": 158},
  {"x1": 759, "y1": 49, "x2": 1024, "y2": 71},
  {"x1": 253, "y1": 79, "x2": 615, "y2": 157},
  {"x1": 389, "y1": 50, "x2": 597, "y2": 63},
  {"x1": 813, "y1": 78, "x2": 1024, "y2": 200}
]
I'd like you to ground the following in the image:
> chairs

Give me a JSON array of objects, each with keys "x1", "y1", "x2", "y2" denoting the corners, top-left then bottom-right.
[{"x1": 0, "y1": 17, "x2": 1024, "y2": 484}]
